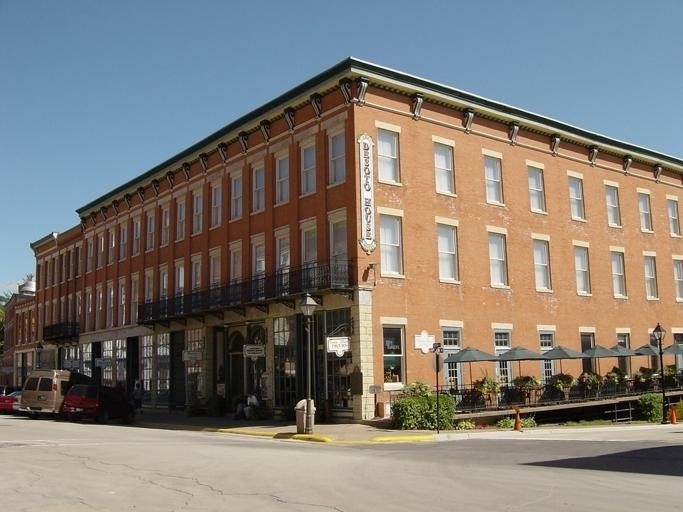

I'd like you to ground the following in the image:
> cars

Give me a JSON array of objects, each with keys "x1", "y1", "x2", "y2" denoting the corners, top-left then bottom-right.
[{"x1": 0, "y1": 384, "x2": 22, "y2": 413}]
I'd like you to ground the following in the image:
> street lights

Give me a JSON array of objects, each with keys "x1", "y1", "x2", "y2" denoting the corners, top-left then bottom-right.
[
  {"x1": 297, "y1": 291, "x2": 316, "y2": 435},
  {"x1": 36, "y1": 341, "x2": 43, "y2": 369},
  {"x1": 652, "y1": 323, "x2": 669, "y2": 424}
]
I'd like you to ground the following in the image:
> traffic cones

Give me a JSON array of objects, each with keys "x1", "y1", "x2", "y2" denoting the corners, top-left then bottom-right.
[
  {"x1": 512, "y1": 408, "x2": 521, "y2": 431},
  {"x1": 668, "y1": 408, "x2": 678, "y2": 425}
]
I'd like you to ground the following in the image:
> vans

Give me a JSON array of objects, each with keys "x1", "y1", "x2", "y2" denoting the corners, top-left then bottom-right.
[{"x1": 18, "y1": 368, "x2": 92, "y2": 416}]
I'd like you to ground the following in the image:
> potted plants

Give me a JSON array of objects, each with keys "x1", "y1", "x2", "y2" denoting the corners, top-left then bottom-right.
[{"x1": 472, "y1": 365, "x2": 683, "y2": 409}]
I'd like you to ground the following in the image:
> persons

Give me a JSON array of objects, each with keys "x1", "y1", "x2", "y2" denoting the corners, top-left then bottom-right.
[
  {"x1": 117, "y1": 376, "x2": 144, "y2": 414},
  {"x1": 232, "y1": 391, "x2": 260, "y2": 420}
]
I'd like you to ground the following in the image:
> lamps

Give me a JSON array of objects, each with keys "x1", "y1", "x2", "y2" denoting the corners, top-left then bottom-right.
[{"x1": 366, "y1": 264, "x2": 378, "y2": 287}]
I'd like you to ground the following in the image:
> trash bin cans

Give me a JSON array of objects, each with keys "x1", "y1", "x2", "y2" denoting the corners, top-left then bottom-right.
[{"x1": 294, "y1": 399, "x2": 317, "y2": 433}]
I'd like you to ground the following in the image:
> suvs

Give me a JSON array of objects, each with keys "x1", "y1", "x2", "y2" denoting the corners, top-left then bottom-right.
[{"x1": 63, "y1": 385, "x2": 135, "y2": 425}]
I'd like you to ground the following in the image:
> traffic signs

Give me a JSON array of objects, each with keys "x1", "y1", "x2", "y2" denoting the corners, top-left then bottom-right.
[{"x1": 428, "y1": 343, "x2": 444, "y2": 353}]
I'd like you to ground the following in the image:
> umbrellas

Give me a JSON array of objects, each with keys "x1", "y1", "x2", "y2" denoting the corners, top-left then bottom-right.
[
  {"x1": 498, "y1": 345, "x2": 550, "y2": 377},
  {"x1": 634, "y1": 343, "x2": 665, "y2": 369},
  {"x1": 609, "y1": 344, "x2": 636, "y2": 379},
  {"x1": 542, "y1": 345, "x2": 591, "y2": 374},
  {"x1": 662, "y1": 343, "x2": 682, "y2": 375},
  {"x1": 583, "y1": 344, "x2": 619, "y2": 375},
  {"x1": 444, "y1": 347, "x2": 503, "y2": 388}
]
